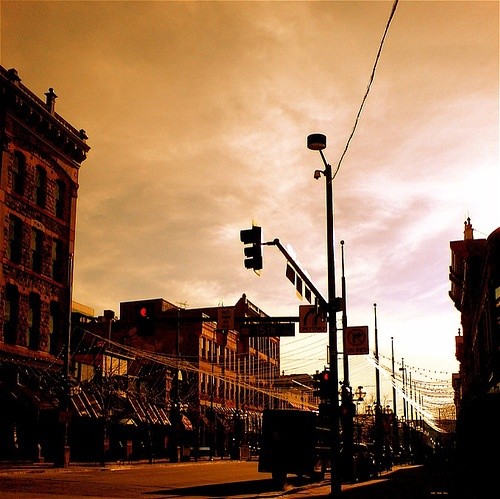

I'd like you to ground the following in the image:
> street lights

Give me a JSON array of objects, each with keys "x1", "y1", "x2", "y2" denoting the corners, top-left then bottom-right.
[{"x1": 306, "y1": 133, "x2": 341, "y2": 497}]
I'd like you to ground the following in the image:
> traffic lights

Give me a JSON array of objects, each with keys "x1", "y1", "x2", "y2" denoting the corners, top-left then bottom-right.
[{"x1": 136, "y1": 302, "x2": 156, "y2": 336}]
